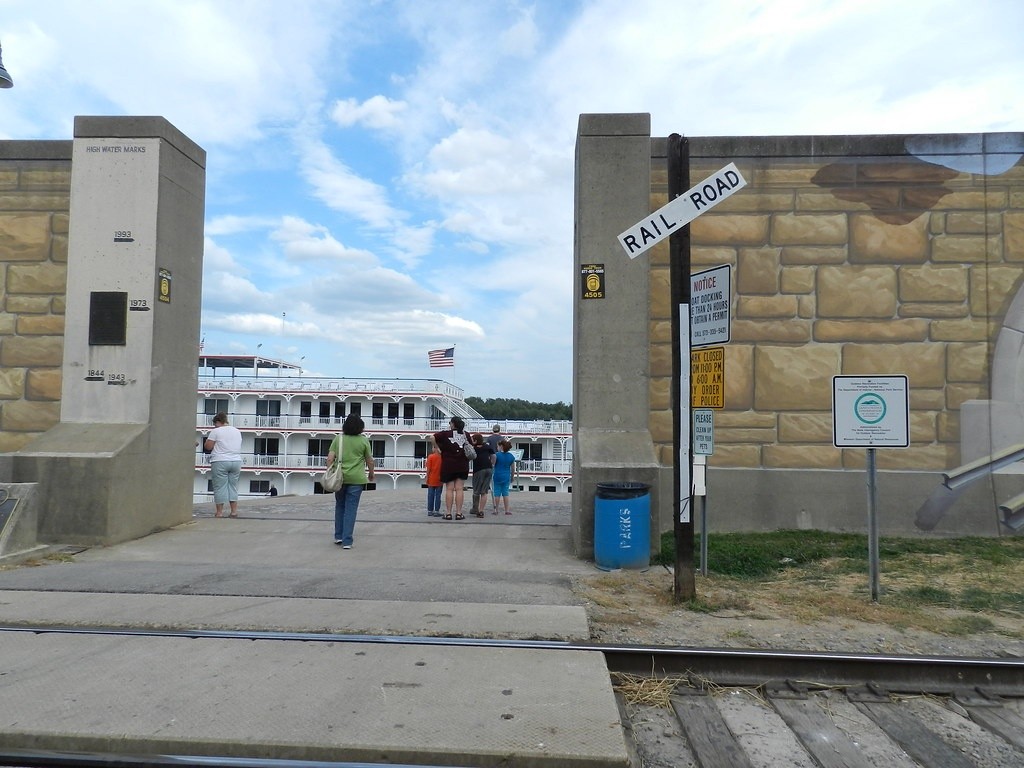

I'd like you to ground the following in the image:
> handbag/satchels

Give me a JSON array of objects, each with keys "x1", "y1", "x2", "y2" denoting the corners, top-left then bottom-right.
[
  {"x1": 321, "y1": 466, "x2": 344, "y2": 493},
  {"x1": 462, "y1": 430, "x2": 477, "y2": 460}
]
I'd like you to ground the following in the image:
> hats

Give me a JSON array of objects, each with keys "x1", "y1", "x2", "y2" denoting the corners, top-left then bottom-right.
[{"x1": 493, "y1": 424, "x2": 500, "y2": 431}]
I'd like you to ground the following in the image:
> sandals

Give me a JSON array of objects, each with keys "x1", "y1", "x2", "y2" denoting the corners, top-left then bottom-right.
[
  {"x1": 442, "y1": 514, "x2": 453, "y2": 520},
  {"x1": 456, "y1": 513, "x2": 465, "y2": 520}
]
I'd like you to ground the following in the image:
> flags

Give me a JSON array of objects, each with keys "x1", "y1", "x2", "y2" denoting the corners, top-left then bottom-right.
[
  {"x1": 428, "y1": 347, "x2": 455, "y2": 368},
  {"x1": 200, "y1": 337, "x2": 206, "y2": 352}
]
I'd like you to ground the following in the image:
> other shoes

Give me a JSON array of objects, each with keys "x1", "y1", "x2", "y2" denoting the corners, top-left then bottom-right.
[
  {"x1": 505, "y1": 512, "x2": 512, "y2": 515},
  {"x1": 333, "y1": 538, "x2": 342, "y2": 544},
  {"x1": 433, "y1": 512, "x2": 444, "y2": 517},
  {"x1": 492, "y1": 512, "x2": 498, "y2": 515},
  {"x1": 476, "y1": 511, "x2": 484, "y2": 518},
  {"x1": 469, "y1": 508, "x2": 478, "y2": 514},
  {"x1": 428, "y1": 511, "x2": 434, "y2": 516},
  {"x1": 342, "y1": 544, "x2": 353, "y2": 549}
]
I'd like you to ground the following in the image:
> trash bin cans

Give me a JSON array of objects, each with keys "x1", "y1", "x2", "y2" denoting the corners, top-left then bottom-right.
[{"x1": 593, "y1": 480, "x2": 653, "y2": 573}]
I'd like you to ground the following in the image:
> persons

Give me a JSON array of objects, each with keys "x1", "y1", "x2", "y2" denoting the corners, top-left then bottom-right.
[
  {"x1": 426, "y1": 441, "x2": 446, "y2": 516},
  {"x1": 431, "y1": 416, "x2": 475, "y2": 520},
  {"x1": 491, "y1": 440, "x2": 515, "y2": 515},
  {"x1": 204, "y1": 412, "x2": 243, "y2": 518},
  {"x1": 469, "y1": 433, "x2": 496, "y2": 517},
  {"x1": 487, "y1": 425, "x2": 505, "y2": 454},
  {"x1": 327, "y1": 413, "x2": 374, "y2": 549}
]
